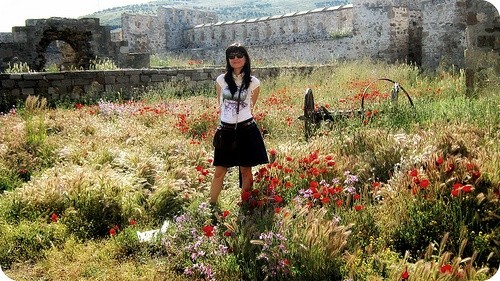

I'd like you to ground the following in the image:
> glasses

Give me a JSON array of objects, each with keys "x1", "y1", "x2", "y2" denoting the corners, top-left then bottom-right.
[{"x1": 227, "y1": 52, "x2": 245, "y2": 58}]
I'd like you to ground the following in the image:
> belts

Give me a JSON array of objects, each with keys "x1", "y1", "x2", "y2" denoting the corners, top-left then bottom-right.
[{"x1": 220, "y1": 119, "x2": 254, "y2": 129}]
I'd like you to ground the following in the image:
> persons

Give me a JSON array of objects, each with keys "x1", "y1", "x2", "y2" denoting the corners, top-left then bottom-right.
[{"x1": 208, "y1": 42, "x2": 268, "y2": 204}]
